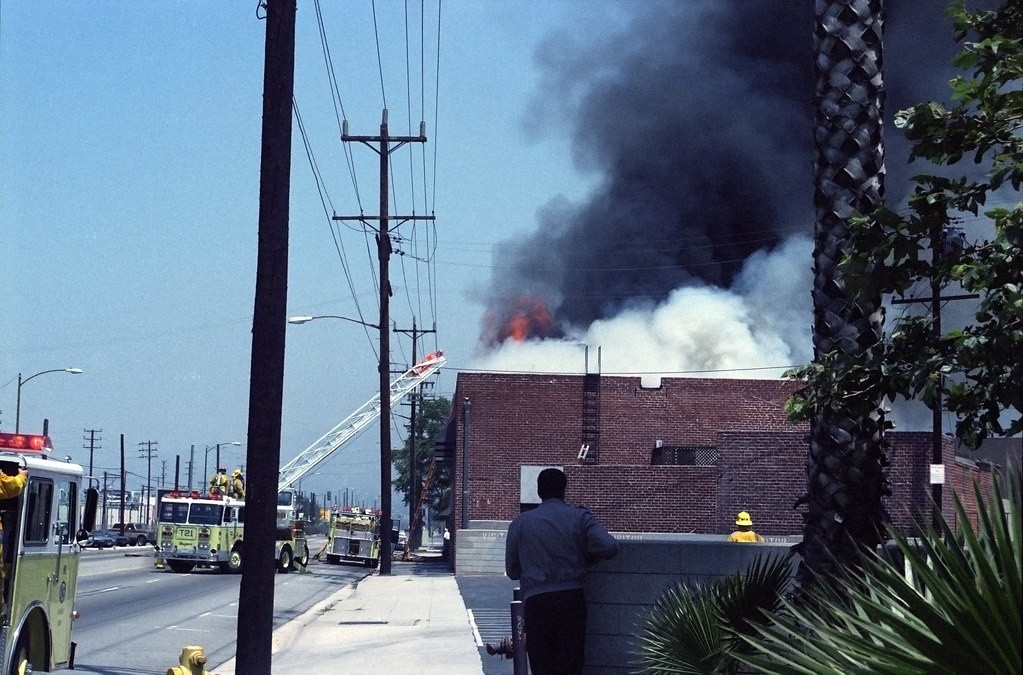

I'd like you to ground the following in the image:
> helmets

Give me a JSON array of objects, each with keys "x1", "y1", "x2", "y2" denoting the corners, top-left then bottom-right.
[
  {"x1": 736, "y1": 511, "x2": 752, "y2": 526},
  {"x1": 231, "y1": 470, "x2": 240, "y2": 476}
]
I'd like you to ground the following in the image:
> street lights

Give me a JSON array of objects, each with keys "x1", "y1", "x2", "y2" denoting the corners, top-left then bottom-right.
[
  {"x1": 204, "y1": 442, "x2": 241, "y2": 493},
  {"x1": 299, "y1": 473, "x2": 321, "y2": 497},
  {"x1": 287, "y1": 314, "x2": 393, "y2": 574},
  {"x1": 16, "y1": 367, "x2": 83, "y2": 435}
]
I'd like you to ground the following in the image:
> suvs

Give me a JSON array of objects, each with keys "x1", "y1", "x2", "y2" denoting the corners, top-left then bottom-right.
[{"x1": 112, "y1": 522, "x2": 155, "y2": 548}]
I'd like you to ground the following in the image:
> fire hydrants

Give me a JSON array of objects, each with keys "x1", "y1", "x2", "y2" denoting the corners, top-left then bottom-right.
[{"x1": 167, "y1": 646, "x2": 216, "y2": 675}]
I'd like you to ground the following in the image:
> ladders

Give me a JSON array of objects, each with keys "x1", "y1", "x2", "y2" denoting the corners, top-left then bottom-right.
[{"x1": 401, "y1": 457, "x2": 436, "y2": 562}]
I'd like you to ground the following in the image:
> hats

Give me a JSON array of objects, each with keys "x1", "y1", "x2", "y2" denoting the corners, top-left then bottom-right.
[{"x1": 218, "y1": 466, "x2": 228, "y2": 471}]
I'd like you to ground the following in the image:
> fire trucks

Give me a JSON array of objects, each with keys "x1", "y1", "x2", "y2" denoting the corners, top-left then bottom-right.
[
  {"x1": 151, "y1": 348, "x2": 447, "y2": 576},
  {"x1": 0, "y1": 433, "x2": 101, "y2": 675},
  {"x1": 324, "y1": 503, "x2": 402, "y2": 568}
]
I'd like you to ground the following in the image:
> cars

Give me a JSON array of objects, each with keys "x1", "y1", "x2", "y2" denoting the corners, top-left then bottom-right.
[{"x1": 80, "y1": 530, "x2": 127, "y2": 549}]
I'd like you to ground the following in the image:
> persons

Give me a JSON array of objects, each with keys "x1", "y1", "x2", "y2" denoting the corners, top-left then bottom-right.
[
  {"x1": 409, "y1": 349, "x2": 444, "y2": 376},
  {"x1": 0, "y1": 468, "x2": 29, "y2": 626},
  {"x1": 505, "y1": 468, "x2": 619, "y2": 675},
  {"x1": 209, "y1": 464, "x2": 245, "y2": 500},
  {"x1": 727, "y1": 511, "x2": 763, "y2": 542}
]
